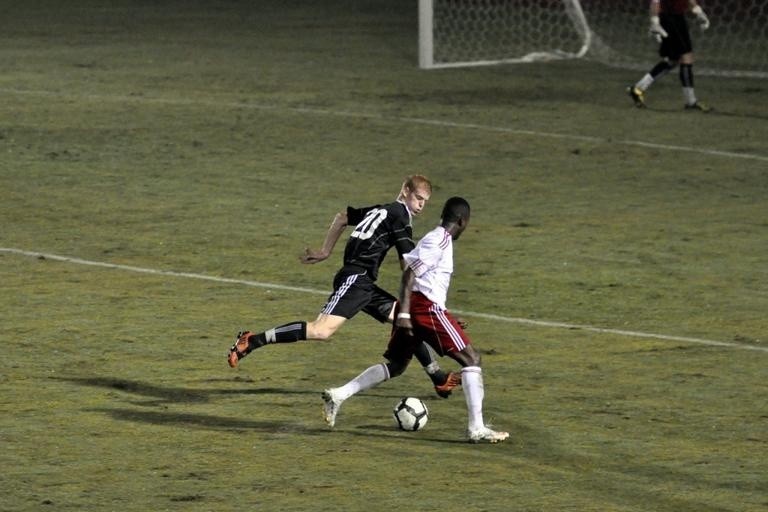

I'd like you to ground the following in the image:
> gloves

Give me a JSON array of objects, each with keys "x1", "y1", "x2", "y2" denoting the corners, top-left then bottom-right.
[
  {"x1": 646, "y1": 23, "x2": 668, "y2": 45},
  {"x1": 694, "y1": 10, "x2": 711, "y2": 30}
]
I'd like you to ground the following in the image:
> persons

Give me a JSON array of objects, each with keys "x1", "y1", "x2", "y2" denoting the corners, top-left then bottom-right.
[
  {"x1": 318, "y1": 196, "x2": 512, "y2": 444},
  {"x1": 226, "y1": 172, "x2": 465, "y2": 403},
  {"x1": 623, "y1": 1, "x2": 714, "y2": 115}
]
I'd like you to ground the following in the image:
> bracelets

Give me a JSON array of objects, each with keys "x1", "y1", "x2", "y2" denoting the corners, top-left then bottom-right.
[{"x1": 396, "y1": 312, "x2": 411, "y2": 321}]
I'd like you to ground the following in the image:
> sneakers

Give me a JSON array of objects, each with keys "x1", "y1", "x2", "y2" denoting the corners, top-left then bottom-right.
[
  {"x1": 468, "y1": 424, "x2": 511, "y2": 445},
  {"x1": 684, "y1": 99, "x2": 714, "y2": 113},
  {"x1": 320, "y1": 386, "x2": 340, "y2": 431},
  {"x1": 225, "y1": 327, "x2": 255, "y2": 369},
  {"x1": 625, "y1": 86, "x2": 650, "y2": 108},
  {"x1": 433, "y1": 368, "x2": 462, "y2": 400}
]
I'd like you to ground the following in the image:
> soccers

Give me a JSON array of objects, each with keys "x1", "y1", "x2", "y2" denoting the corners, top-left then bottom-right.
[{"x1": 393, "y1": 397, "x2": 428, "y2": 431}]
